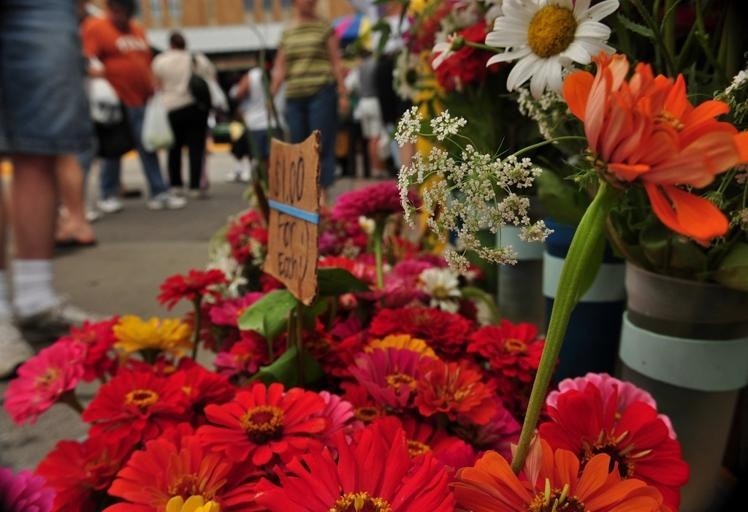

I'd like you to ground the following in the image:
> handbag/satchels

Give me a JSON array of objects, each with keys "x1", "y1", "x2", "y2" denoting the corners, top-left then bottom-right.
[
  {"x1": 89, "y1": 76, "x2": 137, "y2": 162},
  {"x1": 188, "y1": 75, "x2": 211, "y2": 109}
]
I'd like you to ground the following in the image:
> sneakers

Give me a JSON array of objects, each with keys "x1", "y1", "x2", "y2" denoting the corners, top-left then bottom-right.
[
  {"x1": 146, "y1": 187, "x2": 202, "y2": 210},
  {"x1": 0, "y1": 296, "x2": 112, "y2": 380},
  {"x1": 83, "y1": 197, "x2": 123, "y2": 222},
  {"x1": 225, "y1": 171, "x2": 252, "y2": 182}
]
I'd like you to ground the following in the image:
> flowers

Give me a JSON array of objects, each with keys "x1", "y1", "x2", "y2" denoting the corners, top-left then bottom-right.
[{"x1": 1, "y1": 0, "x2": 748, "y2": 512}]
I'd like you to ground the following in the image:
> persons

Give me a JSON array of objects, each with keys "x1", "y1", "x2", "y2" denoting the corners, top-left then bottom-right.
[
  {"x1": 1, "y1": 0, "x2": 88, "y2": 350},
  {"x1": 52, "y1": 2, "x2": 216, "y2": 243},
  {"x1": 230, "y1": 1, "x2": 415, "y2": 221}
]
